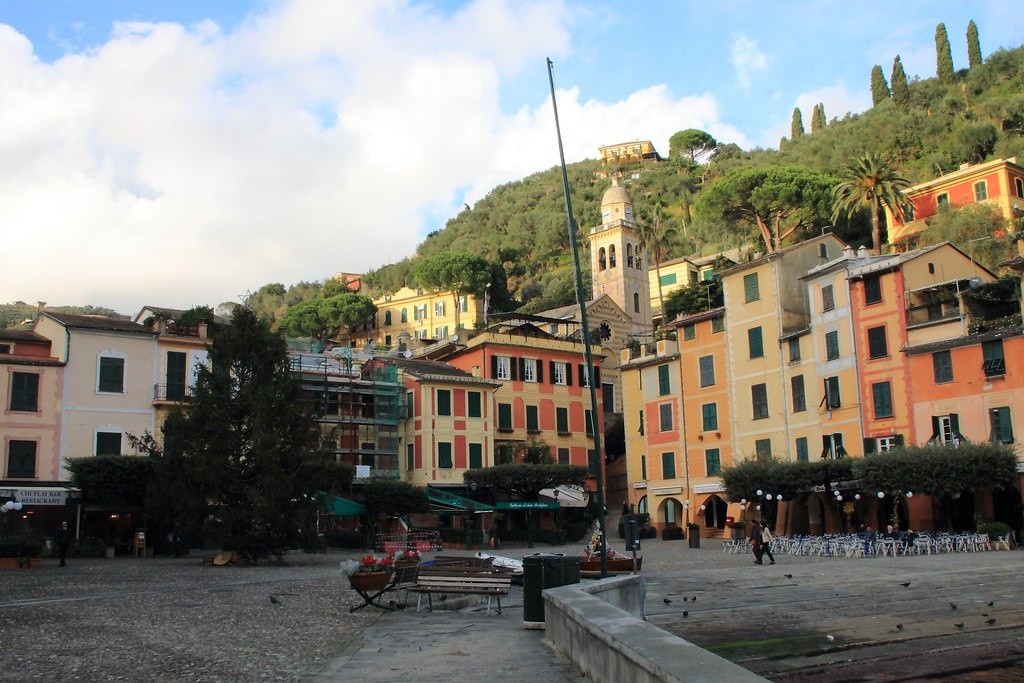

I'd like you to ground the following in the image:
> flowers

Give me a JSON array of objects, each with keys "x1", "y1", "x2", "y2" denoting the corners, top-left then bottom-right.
[{"x1": 337, "y1": 551, "x2": 396, "y2": 576}]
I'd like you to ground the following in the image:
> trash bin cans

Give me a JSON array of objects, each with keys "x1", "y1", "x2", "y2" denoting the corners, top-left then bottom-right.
[{"x1": 521, "y1": 551, "x2": 581, "y2": 630}]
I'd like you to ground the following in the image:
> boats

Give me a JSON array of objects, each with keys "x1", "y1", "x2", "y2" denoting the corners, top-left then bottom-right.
[
  {"x1": 476, "y1": 552, "x2": 567, "y2": 587},
  {"x1": 568, "y1": 520, "x2": 643, "y2": 577}
]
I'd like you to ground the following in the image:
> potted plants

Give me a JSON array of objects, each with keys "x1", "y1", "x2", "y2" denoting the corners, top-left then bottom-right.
[{"x1": 652, "y1": 327, "x2": 676, "y2": 341}]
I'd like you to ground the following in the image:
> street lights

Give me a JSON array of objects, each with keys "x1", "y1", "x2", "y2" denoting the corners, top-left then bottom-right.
[{"x1": 0, "y1": 489, "x2": 28, "y2": 569}]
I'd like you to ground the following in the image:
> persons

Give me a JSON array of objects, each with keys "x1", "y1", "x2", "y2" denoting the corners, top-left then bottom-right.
[
  {"x1": 882, "y1": 525, "x2": 897, "y2": 555},
  {"x1": 592, "y1": 541, "x2": 602, "y2": 558},
  {"x1": 856, "y1": 522, "x2": 866, "y2": 535},
  {"x1": 859, "y1": 525, "x2": 876, "y2": 554},
  {"x1": 749, "y1": 519, "x2": 762, "y2": 564},
  {"x1": 900, "y1": 530, "x2": 913, "y2": 555},
  {"x1": 756, "y1": 520, "x2": 776, "y2": 565},
  {"x1": 53, "y1": 521, "x2": 72, "y2": 567}
]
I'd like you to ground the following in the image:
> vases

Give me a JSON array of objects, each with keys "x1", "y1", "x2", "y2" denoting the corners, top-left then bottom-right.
[
  {"x1": 393, "y1": 560, "x2": 418, "y2": 568},
  {"x1": 338, "y1": 571, "x2": 397, "y2": 591},
  {"x1": 394, "y1": 546, "x2": 422, "y2": 560}
]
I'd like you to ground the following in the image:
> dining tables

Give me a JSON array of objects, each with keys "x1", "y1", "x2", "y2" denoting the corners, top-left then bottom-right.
[{"x1": 718, "y1": 531, "x2": 991, "y2": 558}]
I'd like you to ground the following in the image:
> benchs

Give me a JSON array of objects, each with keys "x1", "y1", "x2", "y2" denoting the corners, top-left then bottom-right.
[
  {"x1": 576, "y1": 554, "x2": 642, "y2": 579},
  {"x1": 407, "y1": 564, "x2": 513, "y2": 615},
  {"x1": 435, "y1": 554, "x2": 493, "y2": 601}
]
